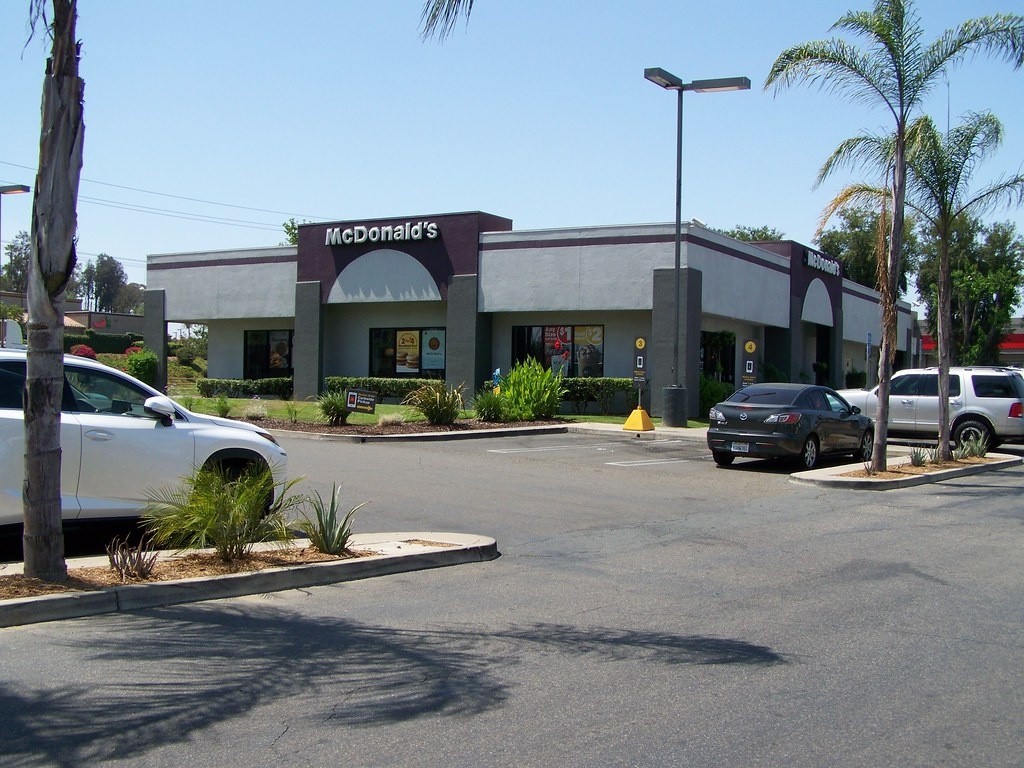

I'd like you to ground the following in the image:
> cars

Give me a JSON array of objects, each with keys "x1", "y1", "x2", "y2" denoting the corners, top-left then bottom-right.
[
  {"x1": 1, "y1": 348, "x2": 288, "y2": 541},
  {"x1": 706, "y1": 383, "x2": 875, "y2": 470}
]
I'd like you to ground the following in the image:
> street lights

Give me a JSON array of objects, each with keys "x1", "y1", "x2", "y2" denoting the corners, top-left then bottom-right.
[
  {"x1": 644, "y1": 68, "x2": 753, "y2": 427},
  {"x1": 1, "y1": 184, "x2": 31, "y2": 341}
]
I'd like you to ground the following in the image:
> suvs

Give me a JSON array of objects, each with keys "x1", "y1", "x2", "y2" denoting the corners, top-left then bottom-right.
[{"x1": 825, "y1": 366, "x2": 1024, "y2": 453}]
